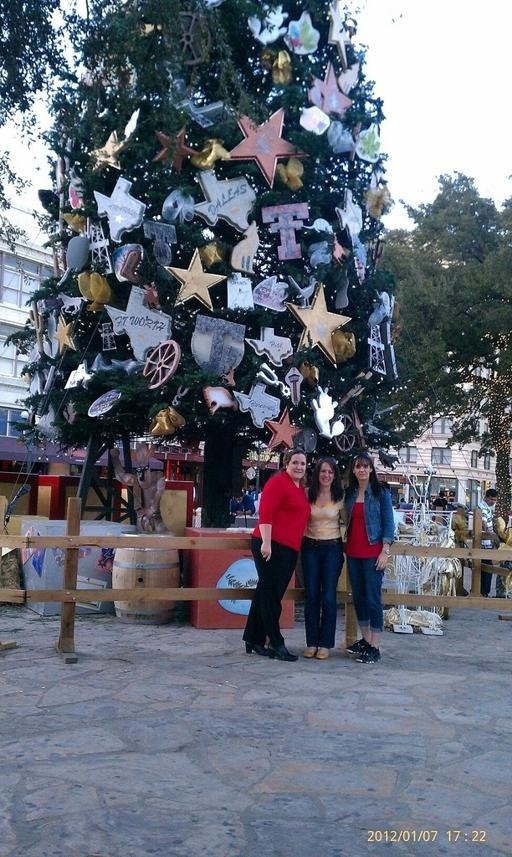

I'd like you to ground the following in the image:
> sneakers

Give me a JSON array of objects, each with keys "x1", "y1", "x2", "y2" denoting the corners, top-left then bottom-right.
[
  {"x1": 346, "y1": 638, "x2": 370, "y2": 654},
  {"x1": 355, "y1": 645, "x2": 382, "y2": 664}
]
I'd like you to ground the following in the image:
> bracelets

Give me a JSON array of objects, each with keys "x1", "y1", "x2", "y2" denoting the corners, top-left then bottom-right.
[{"x1": 381, "y1": 548, "x2": 390, "y2": 555}]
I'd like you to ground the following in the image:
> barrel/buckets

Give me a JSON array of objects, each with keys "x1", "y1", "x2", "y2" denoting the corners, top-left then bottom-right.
[{"x1": 111, "y1": 531, "x2": 179, "y2": 625}]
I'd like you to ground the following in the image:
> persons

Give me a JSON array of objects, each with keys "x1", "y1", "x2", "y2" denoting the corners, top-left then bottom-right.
[
  {"x1": 343, "y1": 453, "x2": 393, "y2": 665},
  {"x1": 396, "y1": 490, "x2": 448, "y2": 524},
  {"x1": 301, "y1": 457, "x2": 346, "y2": 660},
  {"x1": 241, "y1": 449, "x2": 312, "y2": 663},
  {"x1": 477, "y1": 488, "x2": 501, "y2": 596},
  {"x1": 229, "y1": 483, "x2": 263, "y2": 517}
]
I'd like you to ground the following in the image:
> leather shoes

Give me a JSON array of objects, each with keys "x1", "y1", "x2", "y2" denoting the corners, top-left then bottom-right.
[
  {"x1": 316, "y1": 647, "x2": 329, "y2": 659},
  {"x1": 303, "y1": 647, "x2": 316, "y2": 658}
]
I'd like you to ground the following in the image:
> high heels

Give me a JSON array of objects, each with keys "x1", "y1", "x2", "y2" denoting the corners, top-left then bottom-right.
[
  {"x1": 267, "y1": 642, "x2": 298, "y2": 661},
  {"x1": 246, "y1": 641, "x2": 268, "y2": 656}
]
